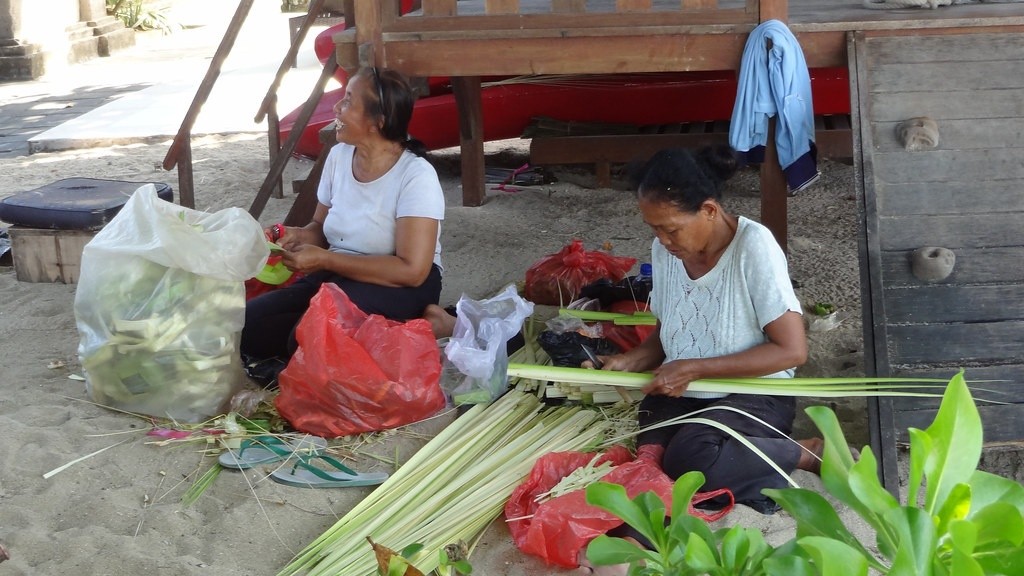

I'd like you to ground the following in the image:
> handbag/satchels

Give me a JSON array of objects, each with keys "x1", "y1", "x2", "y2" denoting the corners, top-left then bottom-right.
[
  {"x1": 500, "y1": 430, "x2": 739, "y2": 568},
  {"x1": 274, "y1": 284, "x2": 452, "y2": 433},
  {"x1": 435, "y1": 277, "x2": 532, "y2": 406},
  {"x1": 73, "y1": 183, "x2": 267, "y2": 420},
  {"x1": 518, "y1": 241, "x2": 638, "y2": 306}
]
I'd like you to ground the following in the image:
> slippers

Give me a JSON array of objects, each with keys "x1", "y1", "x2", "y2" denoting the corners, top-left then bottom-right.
[
  {"x1": 267, "y1": 452, "x2": 390, "y2": 492},
  {"x1": 214, "y1": 434, "x2": 328, "y2": 470}
]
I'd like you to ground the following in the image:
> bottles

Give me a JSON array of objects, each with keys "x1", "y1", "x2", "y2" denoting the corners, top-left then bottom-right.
[{"x1": 631, "y1": 263, "x2": 651, "y2": 302}]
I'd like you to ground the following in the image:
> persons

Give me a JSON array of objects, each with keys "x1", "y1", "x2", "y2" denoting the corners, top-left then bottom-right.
[
  {"x1": 579, "y1": 143, "x2": 862, "y2": 515},
  {"x1": 239, "y1": 65, "x2": 456, "y2": 392}
]
